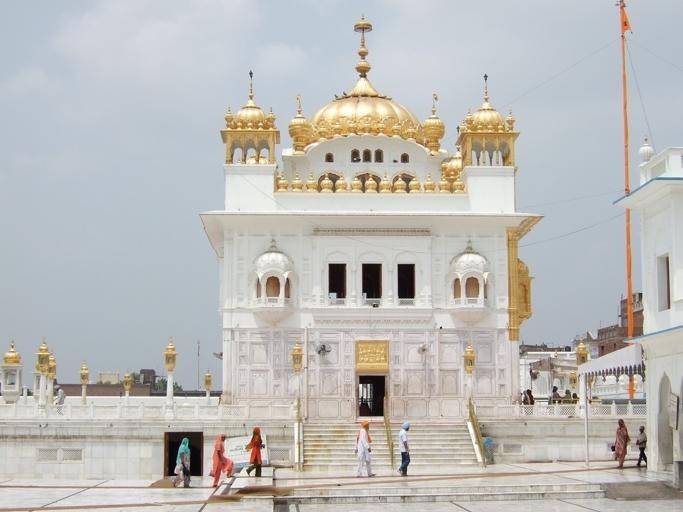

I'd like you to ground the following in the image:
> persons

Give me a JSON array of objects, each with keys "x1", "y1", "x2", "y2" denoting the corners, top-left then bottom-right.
[
  {"x1": 634, "y1": 425, "x2": 646, "y2": 466},
  {"x1": 211, "y1": 433, "x2": 234, "y2": 488},
  {"x1": 395, "y1": 421, "x2": 410, "y2": 477},
  {"x1": 612, "y1": 418, "x2": 627, "y2": 468},
  {"x1": 522, "y1": 385, "x2": 577, "y2": 419},
  {"x1": 355, "y1": 420, "x2": 375, "y2": 477},
  {"x1": 170, "y1": 437, "x2": 191, "y2": 488},
  {"x1": 52, "y1": 384, "x2": 66, "y2": 415},
  {"x1": 244, "y1": 427, "x2": 266, "y2": 478}
]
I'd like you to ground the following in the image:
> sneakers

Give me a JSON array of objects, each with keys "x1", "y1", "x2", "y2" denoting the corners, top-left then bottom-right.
[
  {"x1": 212, "y1": 474, "x2": 233, "y2": 488},
  {"x1": 171, "y1": 480, "x2": 191, "y2": 489},
  {"x1": 245, "y1": 469, "x2": 262, "y2": 478},
  {"x1": 357, "y1": 473, "x2": 376, "y2": 478},
  {"x1": 396, "y1": 469, "x2": 409, "y2": 477}
]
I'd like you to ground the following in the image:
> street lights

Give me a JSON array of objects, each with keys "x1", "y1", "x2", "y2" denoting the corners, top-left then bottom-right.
[
  {"x1": 164, "y1": 342, "x2": 177, "y2": 398},
  {"x1": 80, "y1": 361, "x2": 89, "y2": 404},
  {"x1": 463, "y1": 344, "x2": 476, "y2": 401},
  {"x1": 291, "y1": 341, "x2": 305, "y2": 397},
  {"x1": 123, "y1": 371, "x2": 132, "y2": 403},
  {"x1": 35, "y1": 342, "x2": 50, "y2": 406},
  {"x1": 47, "y1": 353, "x2": 56, "y2": 405},
  {"x1": 204, "y1": 372, "x2": 212, "y2": 397}
]
